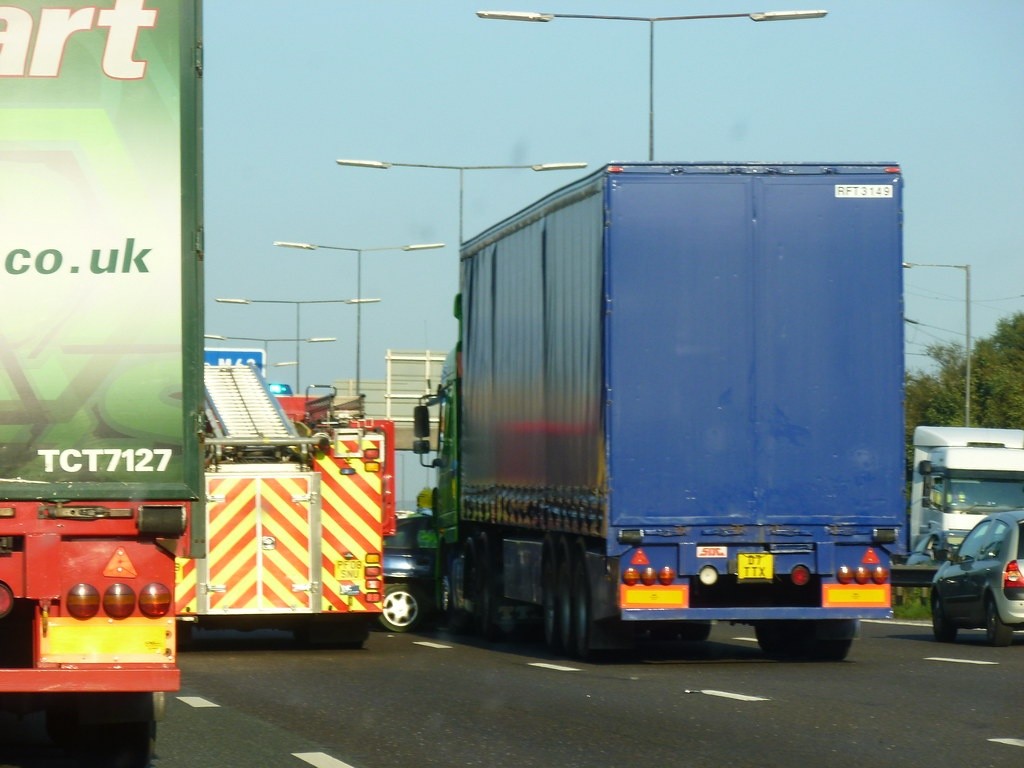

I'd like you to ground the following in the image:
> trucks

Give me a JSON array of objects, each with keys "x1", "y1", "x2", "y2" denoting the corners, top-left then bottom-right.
[
  {"x1": 894, "y1": 423, "x2": 1023, "y2": 566},
  {"x1": 411, "y1": 162, "x2": 908, "y2": 668},
  {"x1": 0, "y1": 0, "x2": 207, "y2": 768}
]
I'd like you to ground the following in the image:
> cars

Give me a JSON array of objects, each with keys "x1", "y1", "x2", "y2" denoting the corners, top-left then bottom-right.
[
  {"x1": 906, "y1": 529, "x2": 970, "y2": 567},
  {"x1": 930, "y1": 511, "x2": 1024, "y2": 648},
  {"x1": 370, "y1": 514, "x2": 441, "y2": 633}
]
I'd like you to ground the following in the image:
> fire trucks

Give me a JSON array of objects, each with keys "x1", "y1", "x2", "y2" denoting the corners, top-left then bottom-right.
[{"x1": 172, "y1": 364, "x2": 396, "y2": 654}]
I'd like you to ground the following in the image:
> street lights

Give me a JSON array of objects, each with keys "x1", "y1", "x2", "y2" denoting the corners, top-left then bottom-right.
[
  {"x1": 476, "y1": 8, "x2": 830, "y2": 161},
  {"x1": 336, "y1": 158, "x2": 590, "y2": 244},
  {"x1": 270, "y1": 240, "x2": 445, "y2": 395},
  {"x1": 204, "y1": 296, "x2": 382, "y2": 393}
]
